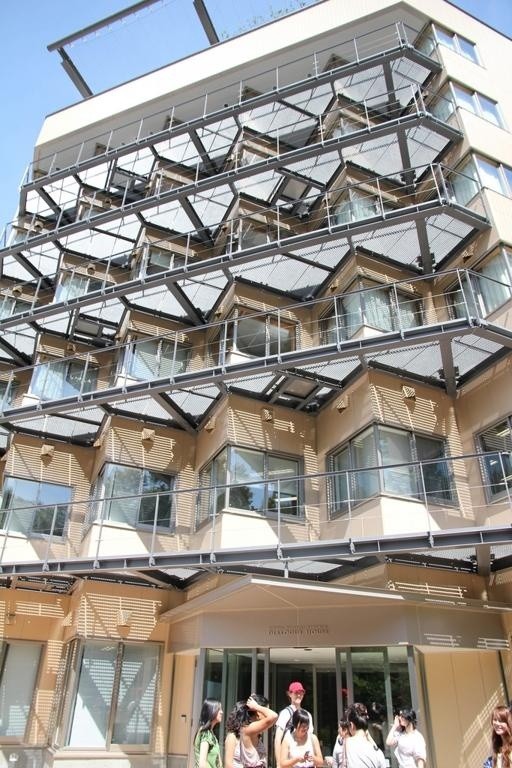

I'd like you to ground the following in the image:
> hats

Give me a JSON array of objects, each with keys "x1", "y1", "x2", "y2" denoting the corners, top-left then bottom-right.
[{"x1": 289, "y1": 681, "x2": 306, "y2": 693}]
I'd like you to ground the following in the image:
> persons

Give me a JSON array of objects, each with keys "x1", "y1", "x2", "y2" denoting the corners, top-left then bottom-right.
[
  {"x1": 343, "y1": 703, "x2": 386, "y2": 768},
  {"x1": 274, "y1": 682, "x2": 314, "y2": 768},
  {"x1": 225, "y1": 732, "x2": 241, "y2": 768},
  {"x1": 489, "y1": 705, "x2": 512, "y2": 768},
  {"x1": 386, "y1": 709, "x2": 427, "y2": 768},
  {"x1": 339, "y1": 719, "x2": 351, "y2": 768},
  {"x1": 227, "y1": 696, "x2": 279, "y2": 768},
  {"x1": 279, "y1": 709, "x2": 323, "y2": 768},
  {"x1": 325, "y1": 733, "x2": 344, "y2": 768},
  {"x1": 193, "y1": 698, "x2": 224, "y2": 768}
]
